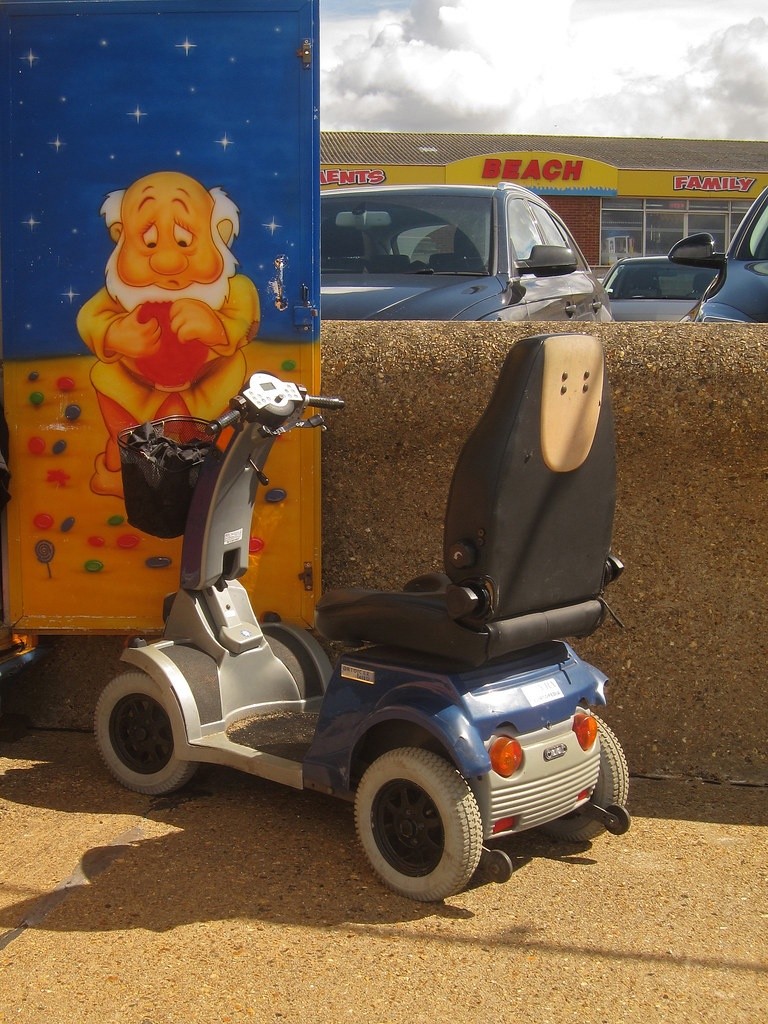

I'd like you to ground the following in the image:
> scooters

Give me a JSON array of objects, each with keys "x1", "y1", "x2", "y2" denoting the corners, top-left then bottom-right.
[{"x1": 93, "y1": 333, "x2": 631, "y2": 900}]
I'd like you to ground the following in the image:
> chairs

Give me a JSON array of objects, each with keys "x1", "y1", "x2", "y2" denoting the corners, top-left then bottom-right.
[
  {"x1": 626, "y1": 271, "x2": 660, "y2": 298},
  {"x1": 687, "y1": 272, "x2": 712, "y2": 297},
  {"x1": 322, "y1": 217, "x2": 364, "y2": 260},
  {"x1": 307, "y1": 328, "x2": 629, "y2": 657},
  {"x1": 437, "y1": 216, "x2": 485, "y2": 266}
]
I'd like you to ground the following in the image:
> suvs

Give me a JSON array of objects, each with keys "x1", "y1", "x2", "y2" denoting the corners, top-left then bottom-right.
[
  {"x1": 320, "y1": 182, "x2": 615, "y2": 322},
  {"x1": 668, "y1": 186, "x2": 768, "y2": 323}
]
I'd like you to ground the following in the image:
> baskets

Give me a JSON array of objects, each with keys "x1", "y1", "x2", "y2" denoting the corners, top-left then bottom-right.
[{"x1": 118, "y1": 416, "x2": 219, "y2": 539}]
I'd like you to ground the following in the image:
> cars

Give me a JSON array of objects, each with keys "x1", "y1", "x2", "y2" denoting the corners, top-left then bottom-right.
[{"x1": 599, "y1": 253, "x2": 720, "y2": 326}]
